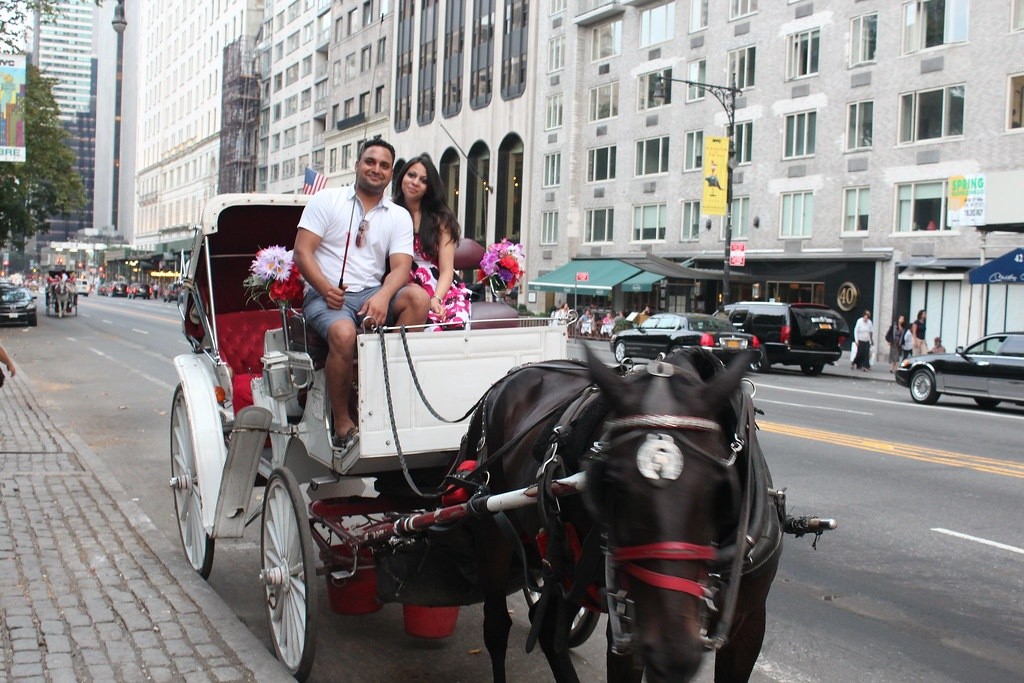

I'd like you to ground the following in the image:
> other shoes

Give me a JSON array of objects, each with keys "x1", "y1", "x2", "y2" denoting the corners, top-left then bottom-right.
[{"x1": 333, "y1": 426, "x2": 359, "y2": 447}]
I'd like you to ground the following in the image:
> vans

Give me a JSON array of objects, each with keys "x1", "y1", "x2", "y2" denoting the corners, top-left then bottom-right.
[{"x1": 75, "y1": 279, "x2": 92, "y2": 296}]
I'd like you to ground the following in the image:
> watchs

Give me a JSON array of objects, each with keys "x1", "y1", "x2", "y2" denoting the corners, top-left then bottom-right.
[{"x1": 433, "y1": 295, "x2": 443, "y2": 304}]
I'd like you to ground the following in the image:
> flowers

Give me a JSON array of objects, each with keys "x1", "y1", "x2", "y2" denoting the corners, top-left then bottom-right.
[
  {"x1": 478, "y1": 238, "x2": 524, "y2": 301},
  {"x1": 246, "y1": 247, "x2": 305, "y2": 305}
]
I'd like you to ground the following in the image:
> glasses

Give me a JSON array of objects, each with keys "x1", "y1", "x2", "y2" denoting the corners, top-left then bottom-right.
[{"x1": 356, "y1": 218, "x2": 370, "y2": 248}]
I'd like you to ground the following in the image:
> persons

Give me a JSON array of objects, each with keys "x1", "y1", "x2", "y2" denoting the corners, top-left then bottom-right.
[
  {"x1": 578, "y1": 310, "x2": 595, "y2": 337},
  {"x1": 390, "y1": 156, "x2": 473, "y2": 332},
  {"x1": 885, "y1": 310, "x2": 946, "y2": 374},
  {"x1": 850, "y1": 311, "x2": 874, "y2": 372},
  {"x1": 863, "y1": 128, "x2": 872, "y2": 146},
  {"x1": 599, "y1": 309, "x2": 625, "y2": 337},
  {"x1": 293, "y1": 138, "x2": 431, "y2": 440},
  {"x1": 549, "y1": 302, "x2": 571, "y2": 343},
  {"x1": 153, "y1": 282, "x2": 159, "y2": 299},
  {"x1": 0, "y1": 345, "x2": 16, "y2": 389},
  {"x1": 47, "y1": 273, "x2": 75, "y2": 286},
  {"x1": 640, "y1": 305, "x2": 657, "y2": 317}
]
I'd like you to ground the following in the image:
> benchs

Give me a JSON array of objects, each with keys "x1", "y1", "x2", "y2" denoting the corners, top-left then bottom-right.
[
  {"x1": 204, "y1": 306, "x2": 304, "y2": 450},
  {"x1": 278, "y1": 238, "x2": 519, "y2": 358}
]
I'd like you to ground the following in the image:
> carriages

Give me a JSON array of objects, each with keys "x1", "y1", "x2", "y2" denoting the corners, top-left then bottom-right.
[
  {"x1": 169, "y1": 191, "x2": 833, "y2": 683},
  {"x1": 45, "y1": 269, "x2": 79, "y2": 318}
]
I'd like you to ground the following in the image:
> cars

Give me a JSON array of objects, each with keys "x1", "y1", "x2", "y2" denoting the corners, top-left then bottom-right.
[
  {"x1": 126, "y1": 282, "x2": 152, "y2": 300},
  {"x1": 99, "y1": 280, "x2": 128, "y2": 296},
  {"x1": 893, "y1": 330, "x2": 1024, "y2": 409},
  {"x1": 607, "y1": 310, "x2": 763, "y2": 369},
  {"x1": 162, "y1": 282, "x2": 180, "y2": 302},
  {"x1": 0, "y1": 286, "x2": 39, "y2": 328}
]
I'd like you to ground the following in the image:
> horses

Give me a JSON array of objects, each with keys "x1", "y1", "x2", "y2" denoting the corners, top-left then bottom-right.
[
  {"x1": 55, "y1": 279, "x2": 69, "y2": 317},
  {"x1": 455, "y1": 340, "x2": 782, "y2": 683}
]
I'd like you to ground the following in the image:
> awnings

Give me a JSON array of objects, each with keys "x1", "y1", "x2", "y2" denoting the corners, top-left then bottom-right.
[
  {"x1": 622, "y1": 260, "x2": 696, "y2": 291},
  {"x1": 966, "y1": 246, "x2": 1024, "y2": 347},
  {"x1": 528, "y1": 259, "x2": 644, "y2": 295}
]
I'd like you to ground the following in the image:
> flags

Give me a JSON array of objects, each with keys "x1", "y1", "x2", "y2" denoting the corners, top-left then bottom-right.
[{"x1": 303, "y1": 168, "x2": 328, "y2": 195}]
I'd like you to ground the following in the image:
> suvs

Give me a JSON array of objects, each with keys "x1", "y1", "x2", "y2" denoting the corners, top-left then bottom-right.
[{"x1": 709, "y1": 301, "x2": 849, "y2": 376}]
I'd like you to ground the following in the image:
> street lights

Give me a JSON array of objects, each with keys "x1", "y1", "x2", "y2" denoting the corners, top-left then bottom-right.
[
  {"x1": 655, "y1": 72, "x2": 744, "y2": 309},
  {"x1": 110, "y1": 0, "x2": 128, "y2": 231}
]
